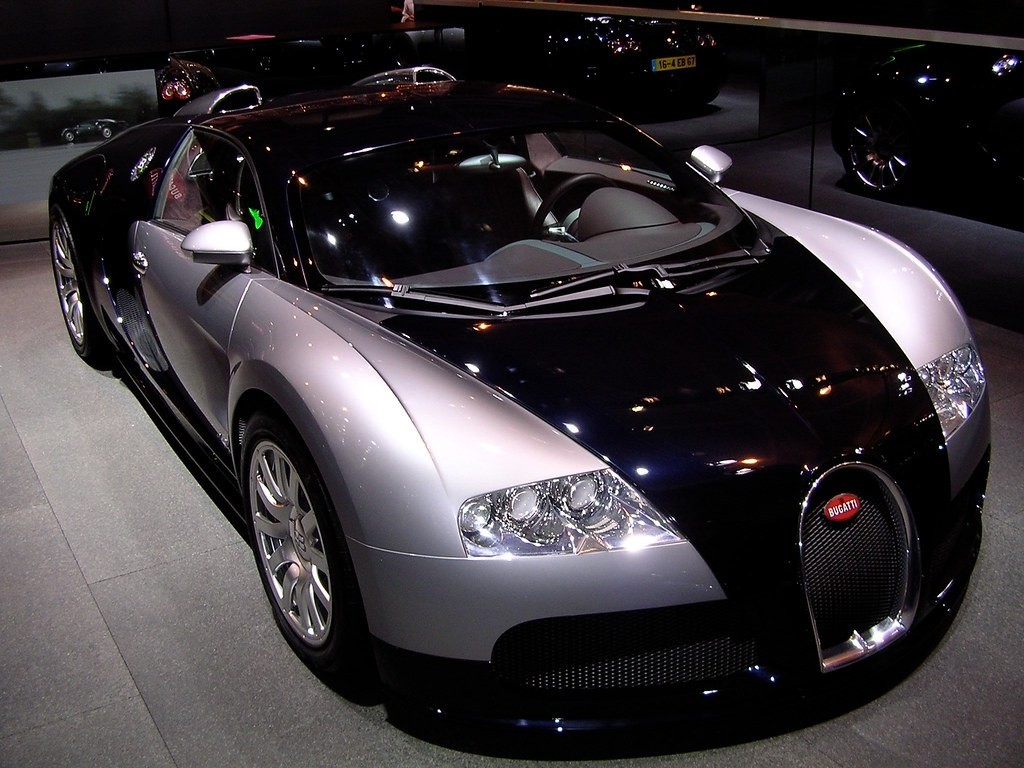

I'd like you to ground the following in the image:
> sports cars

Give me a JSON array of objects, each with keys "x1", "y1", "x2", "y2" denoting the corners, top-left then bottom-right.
[
  {"x1": 830, "y1": 33, "x2": 1024, "y2": 225},
  {"x1": 463, "y1": 0, "x2": 726, "y2": 120},
  {"x1": 48, "y1": 62, "x2": 996, "y2": 742}
]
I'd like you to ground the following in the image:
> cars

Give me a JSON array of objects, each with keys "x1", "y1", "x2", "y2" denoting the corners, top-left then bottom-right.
[{"x1": 59, "y1": 117, "x2": 130, "y2": 144}]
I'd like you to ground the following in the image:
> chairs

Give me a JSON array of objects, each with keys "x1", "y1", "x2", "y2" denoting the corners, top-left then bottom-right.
[{"x1": 443, "y1": 154, "x2": 564, "y2": 264}]
